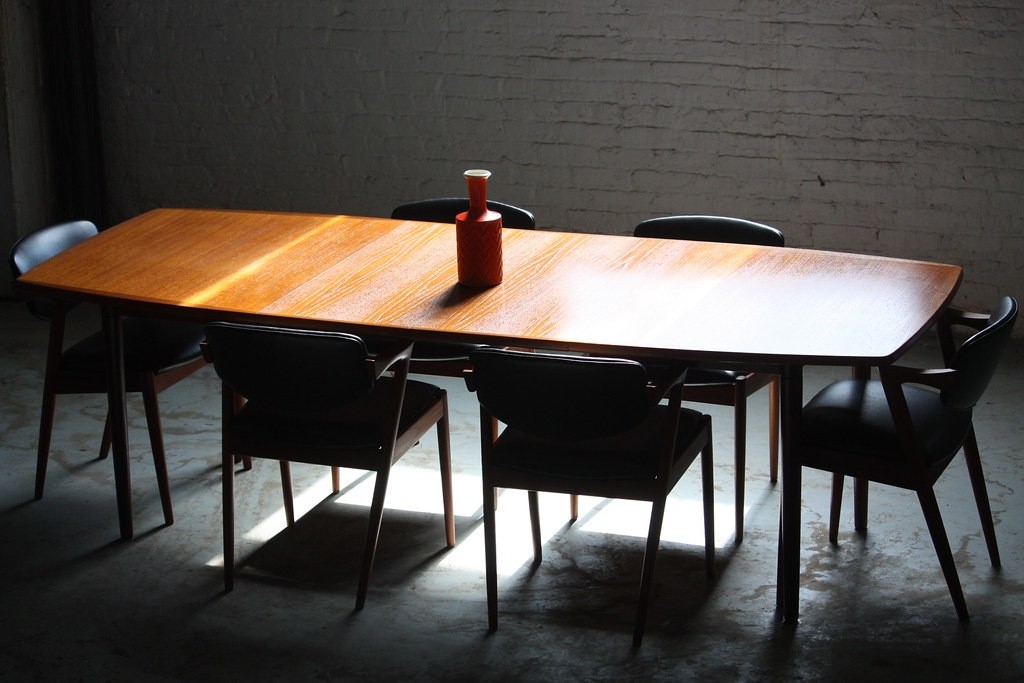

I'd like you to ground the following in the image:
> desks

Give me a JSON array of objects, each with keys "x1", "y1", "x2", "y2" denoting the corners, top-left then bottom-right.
[{"x1": 18, "y1": 208, "x2": 965, "y2": 623}]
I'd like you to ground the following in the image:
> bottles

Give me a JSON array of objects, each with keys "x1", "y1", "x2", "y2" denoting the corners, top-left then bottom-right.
[{"x1": 454, "y1": 168, "x2": 505, "y2": 286}]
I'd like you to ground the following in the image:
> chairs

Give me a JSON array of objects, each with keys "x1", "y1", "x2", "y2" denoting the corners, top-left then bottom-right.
[
  {"x1": 10, "y1": 219, "x2": 254, "y2": 525},
  {"x1": 631, "y1": 215, "x2": 786, "y2": 543},
  {"x1": 199, "y1": 319, "x2": 459, "y2": 610},
  {"x1": 464, "y1": 344, "x2": 716, "y2": 649},
  {"x1": 331, "y1": 197, "x2": 536, "y2": 507},
  {"x1": 802, "y1": 296, "x2": 1020, "y2": 623}
]
